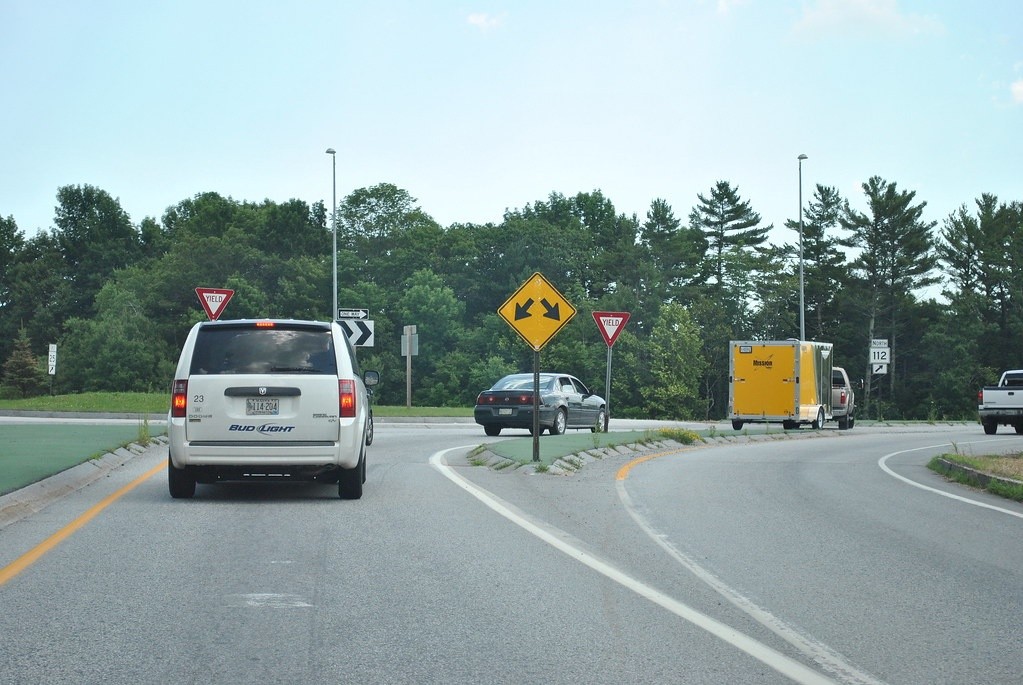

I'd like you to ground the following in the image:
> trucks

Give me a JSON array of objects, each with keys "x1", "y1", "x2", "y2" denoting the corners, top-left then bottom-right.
[{"x1": 724, "y1": 337, "x2": 865, "y2": 430}]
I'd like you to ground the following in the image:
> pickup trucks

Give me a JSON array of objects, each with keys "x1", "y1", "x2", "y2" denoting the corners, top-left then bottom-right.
[{"x1": 978, "y1": 368, "x2": 1023, "y2": 436}]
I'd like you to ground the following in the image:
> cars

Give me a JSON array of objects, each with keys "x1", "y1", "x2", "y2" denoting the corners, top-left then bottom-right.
[{"x1": 473, "y1": 370, "x2": 610, "y2": 436}]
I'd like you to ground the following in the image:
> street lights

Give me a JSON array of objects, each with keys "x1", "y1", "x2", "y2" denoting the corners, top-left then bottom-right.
[
  {"x1": 796, "y1": 153, "x2": 808, "y2": 342},
  {"x1": 325, "y1": 147, "x2": 338, "y2": 324}
]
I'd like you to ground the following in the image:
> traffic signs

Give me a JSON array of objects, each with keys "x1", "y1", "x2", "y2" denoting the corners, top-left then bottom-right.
[
  {"x1": 869, "y1": 338, "x2": 890, "y2": 374},
  {"x1": 337, "y1": 308, "x2": 369, "y2": 320},
  {"x1": 48, "y1": 343, "x2": 58, "y2": 375}
]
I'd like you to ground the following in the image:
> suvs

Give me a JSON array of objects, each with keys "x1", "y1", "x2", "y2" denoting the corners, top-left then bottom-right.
[{"x1": 163, "y1": 317, "x2": 381, "y2": 501}]
